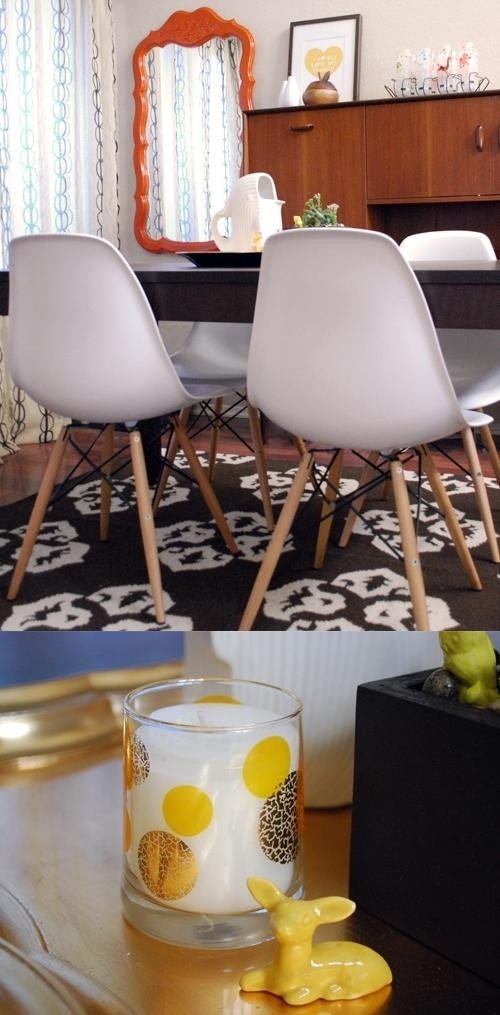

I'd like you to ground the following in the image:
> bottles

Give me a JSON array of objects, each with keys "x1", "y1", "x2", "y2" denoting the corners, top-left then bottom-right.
[{"x1": 278, "y1": 75, "x2": 301, "y2": 107}]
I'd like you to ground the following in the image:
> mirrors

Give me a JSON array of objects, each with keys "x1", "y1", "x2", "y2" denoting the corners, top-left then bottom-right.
[{"x1": 133, "y1": 7, "x2": 255, "y2": 254}]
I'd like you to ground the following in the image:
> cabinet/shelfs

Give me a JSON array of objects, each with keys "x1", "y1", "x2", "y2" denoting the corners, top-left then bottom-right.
[
  {"x1": 242, "y1": 101, "x2": 380, "y2": 232},
  {"x1": 366, "y1": 89, "x2": 500, "y2": 205}
]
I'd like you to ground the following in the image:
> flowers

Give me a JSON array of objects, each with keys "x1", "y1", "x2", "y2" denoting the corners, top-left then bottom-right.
[{"x1": 294, "y1": 193, "x2": 344, "y2": 228}]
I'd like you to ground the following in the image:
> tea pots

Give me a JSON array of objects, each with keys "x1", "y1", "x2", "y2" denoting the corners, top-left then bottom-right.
[{"x1": 211, "y1": 172, "x2": 286, "y2": 252}]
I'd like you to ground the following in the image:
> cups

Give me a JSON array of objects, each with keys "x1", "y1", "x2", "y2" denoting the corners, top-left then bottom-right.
[
  {"x1": 393, "y1": 41, "x2": 478, "y2": 99},
  {"x1": 120, "y1": 677, "x2": 304, "y2": 953}
]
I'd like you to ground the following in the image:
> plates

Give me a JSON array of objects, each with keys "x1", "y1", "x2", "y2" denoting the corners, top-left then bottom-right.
[{"x1": 177, "y1": 252, "x2": 261, "y2": 268}]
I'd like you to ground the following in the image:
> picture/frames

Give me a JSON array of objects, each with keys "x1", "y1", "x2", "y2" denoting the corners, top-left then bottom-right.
[{"x1": 287, "y1": 14, "x2": 363, "y2": 106}]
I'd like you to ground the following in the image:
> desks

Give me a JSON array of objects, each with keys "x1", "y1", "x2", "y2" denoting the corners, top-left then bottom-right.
[{"x1": 1, "y1": 260, "x2": 499, "y2": 486}]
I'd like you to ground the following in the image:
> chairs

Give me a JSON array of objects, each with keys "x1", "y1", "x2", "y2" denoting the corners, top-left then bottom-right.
[
  {"x1": 237, "y1": 229, "x2": 494, "y2": 632},
  {"x1": 340, "y1": 229, "x2": 500, "y2": 547},
  {"x1": 7, "y1": 233, "x2": 237, "y2": 625},
  {"x1": 0, "y1": 630, "x2": 185, "y2": 775},
  {"x1": 151, "y1": 322, "x2": 320, "y2": 533}
]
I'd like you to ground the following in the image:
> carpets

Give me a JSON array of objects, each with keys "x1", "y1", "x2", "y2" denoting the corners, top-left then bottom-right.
[{"x1": 0, "y1": 447, "x2": 500, "y2": 631}]
